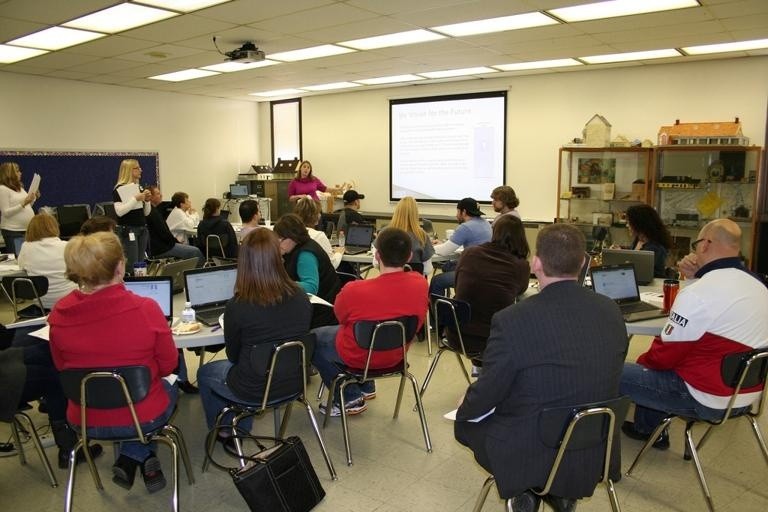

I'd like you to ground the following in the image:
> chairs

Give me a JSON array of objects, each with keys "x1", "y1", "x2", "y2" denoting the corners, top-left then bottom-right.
[
  {"x1": 200, "y1": 231, "x2": 237, "y2": 269},
  {"x1": 471, "y1": 395, "x2": 632, "y2": 512},
  {"x1": 57, "y1": 204, "x2": 92, "y2": 237},
  {"x1": 1, "y1": 348, "x2": 58, "y2": 488},
  {"x1": 59, "y1": 365, "x2": 195, "y2": 512},
  {"x1": 625, "y1": 346, "x2": 768, "y2": 512},
  {"x1": 144, "y1": 247, "x2": 176, "y2": 277},
  {"x1": 404, "y1": 263, "x2": 432, "y2": 354},
  {"x1": 412, "y1": 292, "x2": 482, "y2": 413},
  {"x1": 419, "y1": 218, "x2": 439, "y2": 240},
  {"x1": 318, "y1": 316, "x2": 433, "y2": 467},
  {"x1": 202, "y1": 333, "x2": 339, "y2": 482},
  {"x1": 2, "y1": 276, "x2": 51, "y2": 323}
]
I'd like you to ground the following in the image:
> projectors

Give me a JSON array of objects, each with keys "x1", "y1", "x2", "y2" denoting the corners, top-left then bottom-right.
[{"x1": 232, "y1": 51, "x2": 265, "y2": 63}]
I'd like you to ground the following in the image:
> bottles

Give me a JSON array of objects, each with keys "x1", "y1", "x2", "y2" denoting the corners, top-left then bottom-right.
[
  {"x1": 339, "y1": 231, "x2": 345, "y2": 247},
  {"x1": 182, "y1": 302, "x2": 195, "y2": 323}
]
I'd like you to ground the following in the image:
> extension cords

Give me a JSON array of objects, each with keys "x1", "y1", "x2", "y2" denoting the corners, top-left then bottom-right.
[{"x1": 38, "y1": 433, "x2": 56, "y2": 448}]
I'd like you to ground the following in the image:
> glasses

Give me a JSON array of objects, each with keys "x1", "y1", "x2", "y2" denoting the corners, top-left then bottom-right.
[
  {"x1": 133, "y1": 167, "x2": 142, "y2": 170},
  {"x1": 692, "y1": 239, "x2": 712, "y2": 251},
  {"x1": 278, "y1": 237, "x2": 288, "y2": 242}
]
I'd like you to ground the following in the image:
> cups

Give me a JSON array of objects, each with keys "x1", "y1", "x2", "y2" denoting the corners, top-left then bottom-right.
[{"x1": 133, "y1": 261, "x2": 147, "y2": 277}]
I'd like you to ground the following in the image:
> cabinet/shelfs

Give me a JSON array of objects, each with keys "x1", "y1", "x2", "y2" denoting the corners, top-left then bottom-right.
[
  {"x1": 556, "y1": 147, "x2": 656, "y2": 268},
  {"x1": 651, "y1": 144, "x2": 762, "y2": 282}
]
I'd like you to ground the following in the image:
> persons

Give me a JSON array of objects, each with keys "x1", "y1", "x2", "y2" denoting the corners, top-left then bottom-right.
[
  {"x1": 196, "y1": 227, "x2": 312, "y2": 445},
  {"x1": 447, "y1": 214, "x2": 531, "y2": 378},
  {"x1": 288, "y1": 161, "x2": 342, "y2": 231},
  {"x1": 196, "y1": 198, "x2": 238, "y2": 258},
  {"x1": 17, "y1": 212, "x2": 83, "y2": 310},
  {"x1": 145, "y1": 186, "x2": 204, "y2": 268},
  {"x1": 620, "y1": 219, "x2": 768, "y2": 450},
  {"x1": 0, "y1": 322, "x2": 104, "y2": 469},
  {"x1": 78, "y1": 218, "x2": 199, "y2": 395},
  {"x1": 112, "y1": 159, "x2": 152, "y2": 276},
  {"x1": 371, "y1": 197, "x2": 435, "y2": 277},
  {"x1": 333, "y1": 190, "x2": 376, "y2": 242},
  {"x1": 0, "y1": 161, "x2": 41, "y2": 304},
  {"x1": 292, "y1": 197, "x2": 346, "y2": 270},
  {"x1": 237, "y1": 200, "x2": 263, "y2": 244},
  {"x1": 309, "y1": 228, "x2": 429, "y2": 417},
  {"x1": 490, "y1": 186, "x2": 522, "y2": 228},
  {"x1": 48, "y1": 230, "x2": 180, "y2": 493},
  {"x1": 454, "y1": 221, "x2": 629, "y2": 512},
  {"x1": 165, "y1": 191, "x2": 199, "y2": 246},
  {"x1": 430, "y1": 198, "x2": 494, "y2": 332},
  {"x1": 272, "y1": 213, "x2": 343, "y2": 377},
  {"x1": 610, "y1": 203, "x2": 672, "y2": 277}
]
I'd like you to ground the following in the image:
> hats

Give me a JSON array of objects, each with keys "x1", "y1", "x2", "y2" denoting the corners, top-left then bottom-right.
[
  {"x1": 343, "y1": 190, "x2": 364, "y2": 203},
  {"x1": 457, "y1": 198, "x2": 486, "y2": 216}
]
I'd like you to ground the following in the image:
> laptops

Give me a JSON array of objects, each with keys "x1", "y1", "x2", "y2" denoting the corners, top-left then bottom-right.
[
  {"x1": 14, "y1": 236, "x2": 27, "y2": 260},
  {"x1": 123, "y1": 276, "x2": 173, "y2": 329},
  {"x1": 183, "y1": 264, "x2": 240, "y2": 327},
  {"x1": 230, "y1": 184, "x2": 249, "y2": 198},
  {"x1": 589, "y1": 266, "x2": 670, "y2": 323},
  {"x1": 343, "y1": 224, "x2": 374, "y2": 256},
  {"x1": 602, "y1": 249, "x2": 655, "y2": 286}
]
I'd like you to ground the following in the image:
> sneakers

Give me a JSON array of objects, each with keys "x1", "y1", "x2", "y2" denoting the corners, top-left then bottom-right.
[
  {"x1": 361, "y1": 390, "x2": 376, "y2": 399},
  {"x1": 319, "y1": 396, "x2": 368, "y2": 416}
]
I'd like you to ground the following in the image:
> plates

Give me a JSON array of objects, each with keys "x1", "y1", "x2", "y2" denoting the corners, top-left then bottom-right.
[{"x1": 172, "y1": 322, "x2": 201, "y2": 334}]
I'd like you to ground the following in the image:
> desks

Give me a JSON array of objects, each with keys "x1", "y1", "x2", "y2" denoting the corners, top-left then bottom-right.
[
  {"x1": 27, "y1": 292, "x2": 333, "y2": 348},
  {"x1": 334, "y1": 239, "x2": 461, "y2": 281},
  {"x1": 517, "y1": 278, "x2": 704, "y2": 337},
  {"x1": 176, "y1": 222, "x2": 274, "y2": 245}
]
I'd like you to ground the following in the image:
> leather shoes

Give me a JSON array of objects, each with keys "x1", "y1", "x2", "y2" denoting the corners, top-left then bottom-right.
[
  {"x1": 545, "y1": 493, "x2": 577, "y2": 512},
  {"x1": 622, "y1": 421, "x2": 670, "y2": 450},
  {"x1": 111, "y1": 455, "x2": 136, "y2": 489},
  {"x1": 140, "y1": 452, "x2": 166, "y2": 493},
  {"x1": 506, "y1": 487, "x2": 537, "y2": 512},
  {"x1": 58, "y1": 443, "x2": 102, "y2": 469},
  {"x1": 214, "y1": 430, "x2": 243, "y2": 448}
]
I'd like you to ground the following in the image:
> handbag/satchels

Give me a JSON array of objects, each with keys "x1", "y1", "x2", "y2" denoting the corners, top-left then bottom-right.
[{"x1": 206, "y1": 426, "x2": 325, "y2": 512}]
